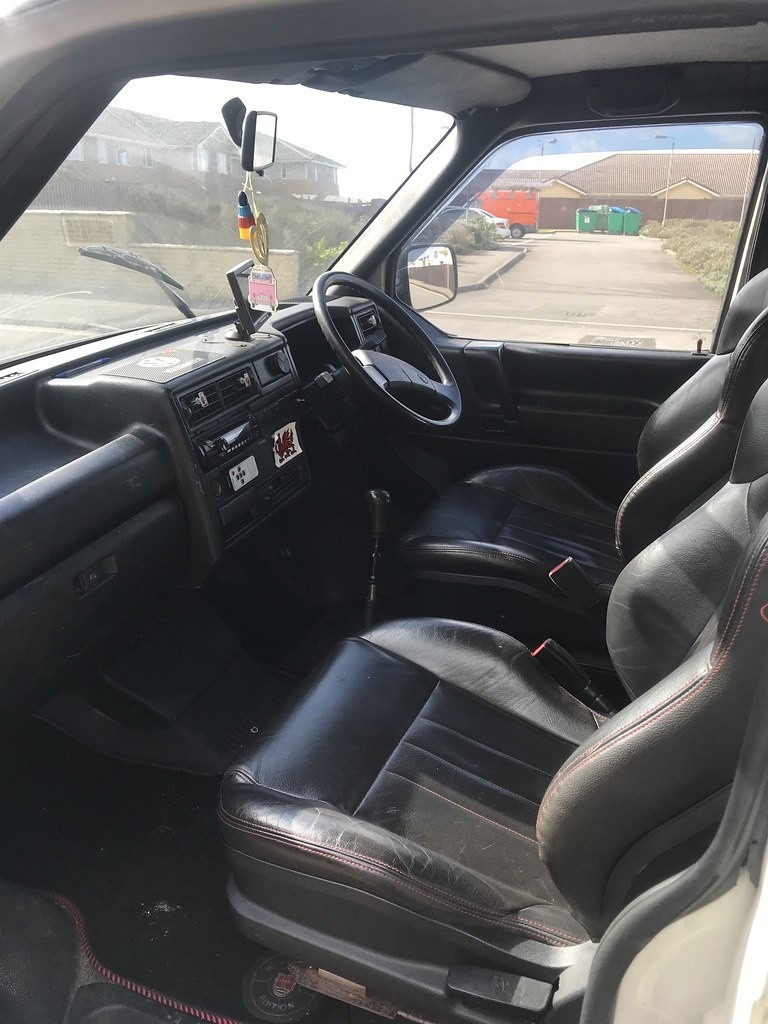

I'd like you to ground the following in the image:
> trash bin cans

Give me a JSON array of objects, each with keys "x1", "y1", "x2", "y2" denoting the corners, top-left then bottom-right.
[{"x1": 576, "y1": 204, "x2": 642, "y2": 235}]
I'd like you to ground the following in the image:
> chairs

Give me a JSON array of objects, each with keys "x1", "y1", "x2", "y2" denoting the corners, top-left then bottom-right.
[
  {"x1": 209, "y1": 373, "x2": 768, "y2": 1023},
  {"x1": 388, "y1": 261, "x2": 767, "y2": 706}
]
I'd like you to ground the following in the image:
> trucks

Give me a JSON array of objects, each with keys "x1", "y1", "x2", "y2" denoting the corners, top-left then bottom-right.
[{"x1": 462, "y1": 189, "x2": 538, "y2": 239}]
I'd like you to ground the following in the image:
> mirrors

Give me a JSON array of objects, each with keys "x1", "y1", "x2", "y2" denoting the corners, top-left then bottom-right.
[{"x1": 220, "y1": 96, "x2": 277, "y2": 172}]
